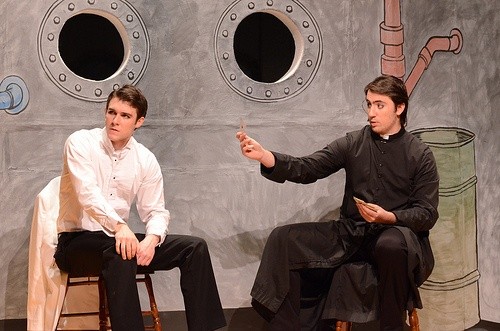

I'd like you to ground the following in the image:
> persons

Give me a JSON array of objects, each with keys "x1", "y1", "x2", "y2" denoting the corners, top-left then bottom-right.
[
  {"x1": 55, "y1": 86, "x2": 227, "y2": 331},
  {"x1": 236, "y1": 75, "x2": 439, "y2": 331}
]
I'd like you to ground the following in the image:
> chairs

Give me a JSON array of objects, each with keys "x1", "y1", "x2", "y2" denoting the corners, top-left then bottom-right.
[{"x1": 27, "y1": 171, "x2": 162, "y2": 330}]
